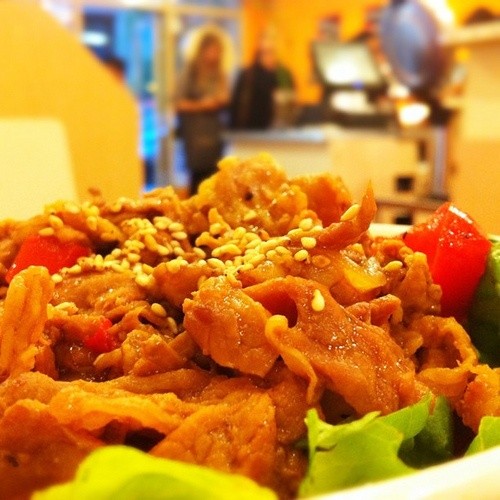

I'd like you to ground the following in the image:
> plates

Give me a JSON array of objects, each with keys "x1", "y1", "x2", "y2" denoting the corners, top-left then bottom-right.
[{"x1": 293, "y1": 218, "x2": 498, "y2": 500}]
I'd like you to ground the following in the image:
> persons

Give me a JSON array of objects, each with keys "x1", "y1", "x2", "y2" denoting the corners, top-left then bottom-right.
[
  {"x1": 357, "y1": 28, "x2": 387, "y2": 68},
  {"x1": 102, "y1": 54, "x2": 127, "y2": 80},
  {"x1": 264, "y1": 46, "x2": 297, "y2": 125},
  {"x1": 171, "y1": 32, "x2": 234, "y2": 198},
  {"x1": 227, "y1": 46, "x2": 275, "y2": 131}
]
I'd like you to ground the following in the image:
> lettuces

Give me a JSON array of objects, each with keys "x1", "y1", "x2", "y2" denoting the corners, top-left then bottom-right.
[{"x1": 27, "y1": 393, "x2": 500, "y2": 500}]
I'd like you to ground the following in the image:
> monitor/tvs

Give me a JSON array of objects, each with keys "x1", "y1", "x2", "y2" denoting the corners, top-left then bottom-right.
[{"x1": 312, "y1": 39, "x2": 388, "y2": 92}]
